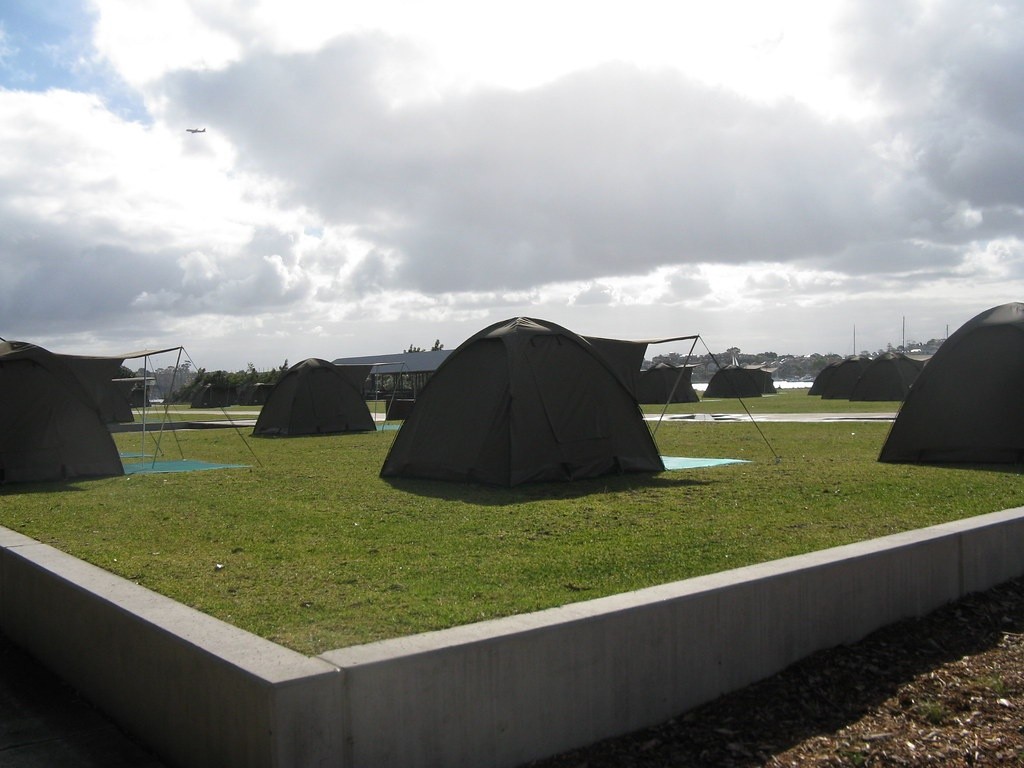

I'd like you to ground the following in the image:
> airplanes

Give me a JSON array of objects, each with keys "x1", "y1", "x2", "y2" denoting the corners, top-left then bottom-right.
[{"x1": 185, "y1": 128, "x2": 207, "y2": 135}]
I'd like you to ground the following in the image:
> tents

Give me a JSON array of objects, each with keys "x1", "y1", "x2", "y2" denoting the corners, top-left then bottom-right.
[{"x1": 0, "y1": 301, "x2": 1024, "y2": 484}]
